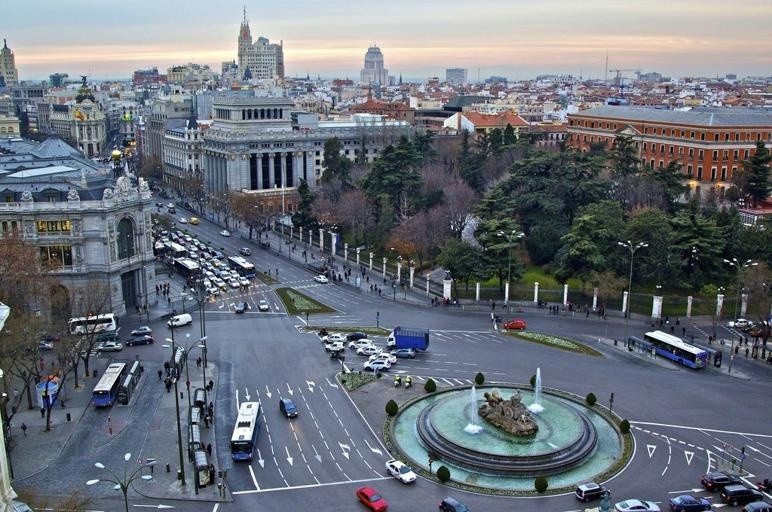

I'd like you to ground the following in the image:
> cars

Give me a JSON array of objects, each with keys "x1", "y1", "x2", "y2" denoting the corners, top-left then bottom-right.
[
  {"x1": 313, "y1": 274, "x2": 328, "y2": 284},
  {"x1": 613, "y1": 469, "x2": 771, "y2": 512},
  {"x1": 504, "y1": 320, "x2": 527, "y2": 330},
  {"x1": 152, "y1": 201, "x2": 256, "y2": 295},
  {"x1": 258, "y1": 300, "x2": 269, "y2": 311},
  {"x1": 95, "y1": 325, "x2": 156, "y2": 352},
  {"x1": 727, "y1": 319, "x2": 772, "y2": 337},
  {"x1": 38, "y1": 335, "x2": 61, "y2": 350},
  {"x1": 439, "y1": 497, "x2": 470, "y2": 512},
  {"x1": 385, "y1": 459, "x2": 417, "y2": 484},
  {"x1": 356, "y1": 486, "x2": 389, "y2": 512},
  {"x1": 318, "y1": 331, "x2": 416, "y2": 372},
  {"x1": 279, "y1": 398, "x2": 299, "y2": 418},
  {"x1": 574, "y1": 482, "x2": 611, "y2": 501},
  {"x1": 235, "y1": 302, "x2": 248, "y2": 314}
]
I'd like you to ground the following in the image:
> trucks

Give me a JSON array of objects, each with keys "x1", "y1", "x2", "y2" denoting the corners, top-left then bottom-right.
[{"x1": 387, "y1": 326, "x2": 430, "y2": 350}]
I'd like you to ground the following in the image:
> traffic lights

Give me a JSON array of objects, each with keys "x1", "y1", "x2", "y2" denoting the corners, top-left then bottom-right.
[{"x1": 218, "y1": 470, "x2": 228, "y2": 479}]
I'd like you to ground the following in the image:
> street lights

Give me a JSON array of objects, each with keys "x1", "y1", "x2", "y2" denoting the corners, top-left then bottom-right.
[
  {"x1": 741, "y1": 286, "x2": 749, "y2": 295},
  {"x1": 444, "y1": 270, "x2": 451, "y2": 280},
  {"x1": 180, "y1": 279, "x2": 223, "y2": 368},
  {"x1": 717, "y1": 286, "x2": 725, "y2": 294},
  {"x1": 410, "y1": 260, "x2": 415, "y2": 267},
  {"x1": 393, "y1": 284, "x2": 396, "y2": 302},
  {"x1": 656, "y1": 285, "x2": 661, "y2": 296},
  {"x1": 738, "y1": 445, "x2": 746, "y2": 474},
  {"x1": 161, "y1": 332, "x2": 209, "y2": 495},
  {"x1": 397, "y1": 256, "x2": 403, "y2": 263},
  {"x1": 331, "y1": 224, "x2": 338, "y2": 233},
  {"x1": 86, "y1": 453, "x2": 158, "y2": 512},
  {"x1": 723, "y1": 258, "x2": 758, "y2": 373},
  {"x1": 428, "y1": 450, "x2": 434, "y2": 476},
  {"x1": 318, "y1": 220, "x2": 325, "y2": 229},
  {"x1": 609, "y1": 393, "x2": 615, "y2": 413},
  {"x1": 497, "y1": 230, "x2": 525, "y2": 311},
  {"x1": 618, "y1": 240, "x2": 649, "y2": 346}
]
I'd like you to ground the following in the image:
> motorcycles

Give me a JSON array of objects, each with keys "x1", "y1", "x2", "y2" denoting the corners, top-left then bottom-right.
[{"x1": 394, "y1": 380, "x2": 411, "y2": 388}]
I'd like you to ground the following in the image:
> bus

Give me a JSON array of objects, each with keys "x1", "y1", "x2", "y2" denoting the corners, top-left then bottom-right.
[
  {"x1": 67, "y1": 313, "x2": 118, "y2": 336},
  {"x1": 228, "y1": 401, "x2": 261, "y2": 463},
  {"x1": 92, "y1": 363, "x2": 128, "y2": 407},
  {"x1": 643, "y1": 330, "x2": 707, "y2": 370}
]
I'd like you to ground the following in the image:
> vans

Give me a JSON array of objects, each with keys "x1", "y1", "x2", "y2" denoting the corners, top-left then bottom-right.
[{"x1": 167, "y1": 313, "x2": 192, "y2": 327}]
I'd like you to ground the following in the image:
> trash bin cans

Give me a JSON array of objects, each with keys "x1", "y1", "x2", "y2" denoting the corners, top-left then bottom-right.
[
  {"x1": 178, "y1": 470, "x2": 182, "y2": 480},
  {"x1": 93, "y1": 369, "x2": 98, "y2": 377},
  {"x1": 67, "y1": 413, "x2": 71, "y2": 421},
  {"x1": 166, "y1": 463, "x2": 170, "y2": 472}
]
